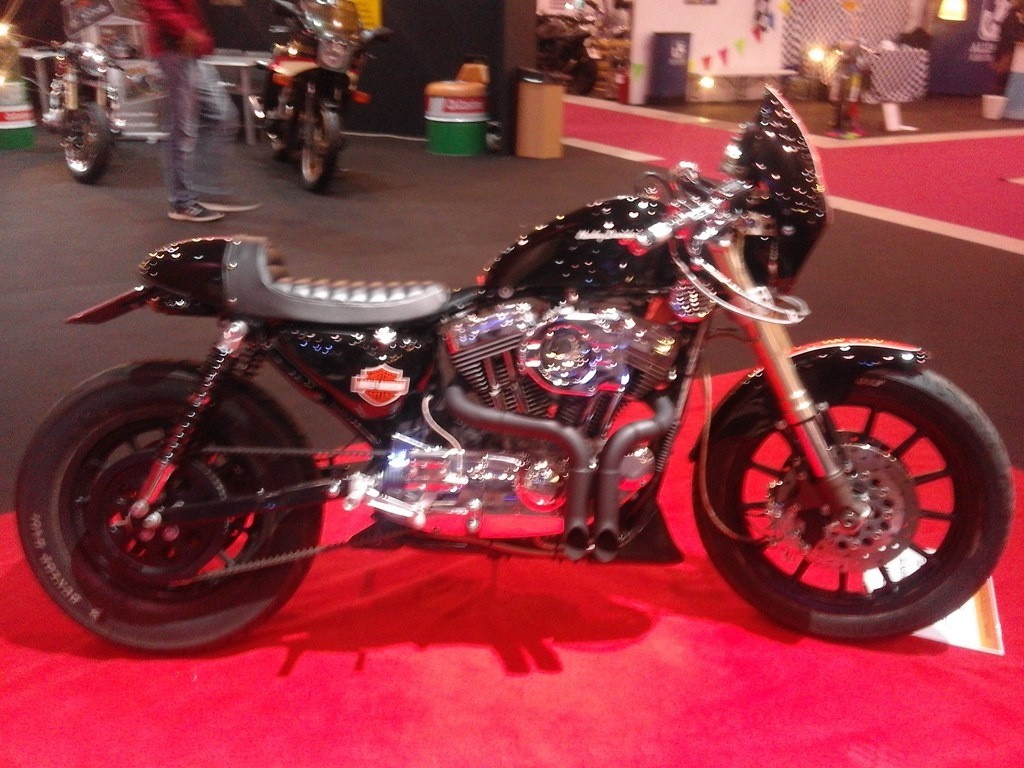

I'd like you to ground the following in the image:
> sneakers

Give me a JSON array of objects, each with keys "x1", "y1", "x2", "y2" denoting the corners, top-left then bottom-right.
[{"x1": 165, "y1": 199, "x2": 223, "y2": 224}]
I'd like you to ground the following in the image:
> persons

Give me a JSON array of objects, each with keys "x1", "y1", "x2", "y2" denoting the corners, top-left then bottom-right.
[{"x1": 131, "y1": 0, "x2": 261, "y2": 222}]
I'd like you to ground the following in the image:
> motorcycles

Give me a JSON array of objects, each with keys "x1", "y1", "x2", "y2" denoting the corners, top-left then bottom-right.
[
  {"x1": 37, "y1": 25, "x2": 127, "y2": 183},
  {"x1": 13, "y1": 83, "x2": 1017, "y2": 647},
  {"x1": 245, "y1": 0, "x2": 391, "y2": 185}
]
